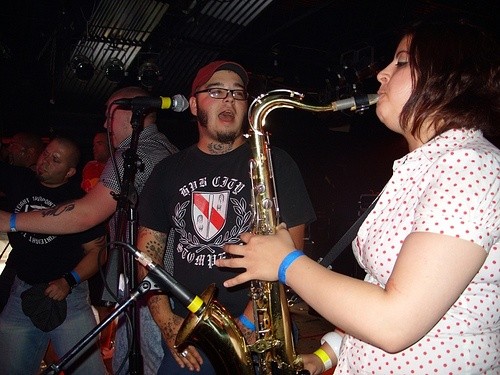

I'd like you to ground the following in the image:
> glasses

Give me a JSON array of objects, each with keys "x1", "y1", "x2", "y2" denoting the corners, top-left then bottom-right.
[{"x1": 193, "y1": 86, "x2": 251, "y2": 102}]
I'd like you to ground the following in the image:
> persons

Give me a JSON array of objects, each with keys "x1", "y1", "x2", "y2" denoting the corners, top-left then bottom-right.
[
  {"x1": 0, "y1": 87, "x2": 180, "y2": 375},
  {"x1": 136, "y1": 61, "x2": 315, "y2": 375},
  {"x1": 0, "y1": 129, "x2": 115, "y2": 375},
  {"x1": 216, "y1": 22, "x2": 500, "y2": 375}
]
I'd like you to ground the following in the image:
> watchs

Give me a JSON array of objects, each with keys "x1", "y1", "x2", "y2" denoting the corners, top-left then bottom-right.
[{"x1": 64, "y1": 272, "x2": 78, "y2": 288}]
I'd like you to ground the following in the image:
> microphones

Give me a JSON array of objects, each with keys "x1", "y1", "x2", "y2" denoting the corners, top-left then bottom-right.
[
  {"x1": 123, "y1": 243, "x2": 206, "y2": 318},
  {"x1": 113, "y1": 95, "x2": 189, "y2": 112}
]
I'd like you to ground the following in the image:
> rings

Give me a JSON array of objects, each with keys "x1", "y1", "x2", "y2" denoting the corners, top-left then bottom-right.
[
  {"x1": 56, "y1": 293, "x2": 59, "y2": 299},
  {"x1": 181, "y1": 349, "x2": 189, "y2": 357}
]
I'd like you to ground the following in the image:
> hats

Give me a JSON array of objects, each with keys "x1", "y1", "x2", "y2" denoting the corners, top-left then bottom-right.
[{"x1": 191, "y1": 60, "x2": 250, "y2": 95}]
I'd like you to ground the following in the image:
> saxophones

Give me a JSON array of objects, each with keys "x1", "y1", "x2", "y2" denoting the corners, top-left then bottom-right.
[{"x1": 173, "y1": 87, "x2": 389, "y2": 375}]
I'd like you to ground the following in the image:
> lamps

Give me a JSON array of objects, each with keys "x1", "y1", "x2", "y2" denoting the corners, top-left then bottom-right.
[
  {"x1": 68, "y1": 44, "x2": 95, "y2": 81},
  {"x1": 103, "y1": 47, "x2": 126, "y2": 83},
  {"x1": 137, "y1": 51, "x2": 161, "y2": 89}
]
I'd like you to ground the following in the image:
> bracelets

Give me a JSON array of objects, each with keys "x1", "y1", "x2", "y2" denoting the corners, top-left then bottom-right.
[
  {"x1": 71, "y1": 271, "x2": 80, "y2": 285},
  {"x1": 63, "y1": 275, "x2": 73, "y2": 289},
  {"x1": 314, "y1": 349, "x2": 332, "y2": 371},
  {"x1": 278, "y1": 250, "x2": 304, "y2": 283},
  {"x1": 10, "y1": 213, "x2": 17, "y2": 232},
  {"x1": 240, "y1": 316, "x2": 255, "y2": 332}
]
